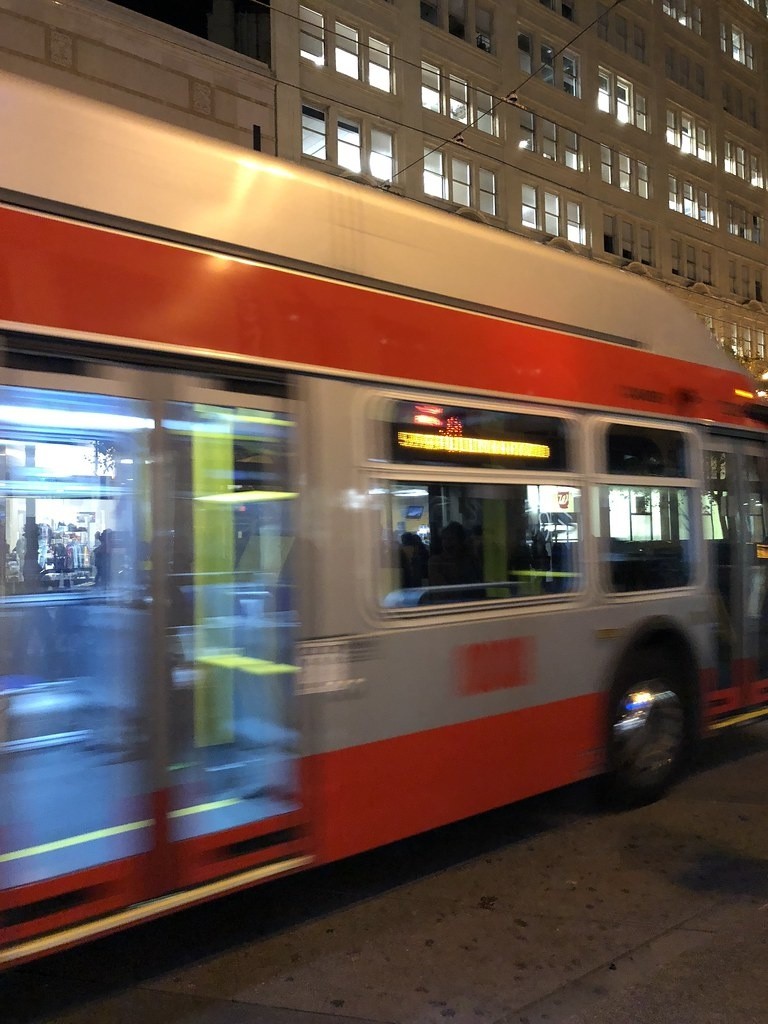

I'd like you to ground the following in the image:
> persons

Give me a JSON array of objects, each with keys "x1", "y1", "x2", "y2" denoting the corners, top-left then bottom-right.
[
  {"x1": 528, "y1": 522, "x2": 551, "y2": 586},
  {"x1": 400, "y1": 521, "x2": 466, "y2": 584},
  {"x1": 95, "y1": 529, "x2": 115, "y2": 593}
]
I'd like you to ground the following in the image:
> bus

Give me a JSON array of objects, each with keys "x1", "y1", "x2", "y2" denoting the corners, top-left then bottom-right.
[{"x1": 1, "y1": 71, "x2": 768, "y2": 968}]
